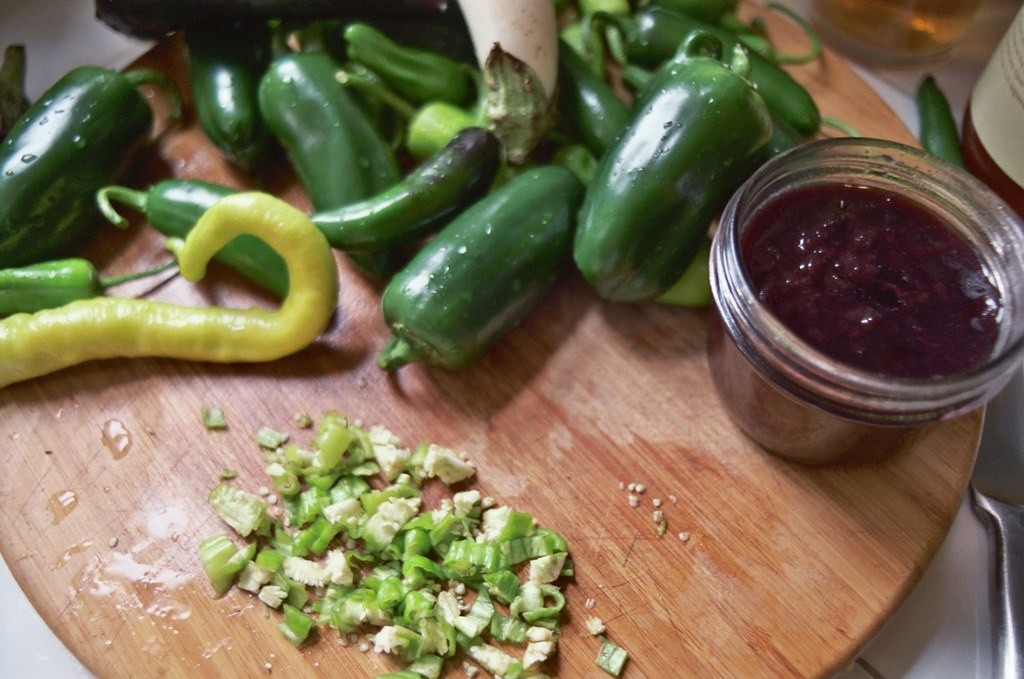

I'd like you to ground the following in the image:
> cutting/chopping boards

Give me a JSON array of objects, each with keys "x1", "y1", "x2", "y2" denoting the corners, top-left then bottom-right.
[{"x1": 1, "y1": 1, "x2": 988, "y2": 679}]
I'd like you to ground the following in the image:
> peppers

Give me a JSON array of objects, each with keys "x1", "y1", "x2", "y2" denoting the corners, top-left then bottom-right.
[
  {"x1": 0, "y1": 0, "x2": 977, "y2": 387},
  {"x1": 199, "y1": 406, "x2": 627, "y2": 679}
]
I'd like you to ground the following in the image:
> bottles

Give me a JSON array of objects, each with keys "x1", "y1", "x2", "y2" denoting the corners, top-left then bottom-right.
[{"x1": 961, "y1": 5, "x2": 1023, "y2": 215}]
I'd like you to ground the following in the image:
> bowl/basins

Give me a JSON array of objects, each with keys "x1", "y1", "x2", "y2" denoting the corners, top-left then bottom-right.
[{"x1": 708, "y1": 138, "x2": 1024, "y2": 464}]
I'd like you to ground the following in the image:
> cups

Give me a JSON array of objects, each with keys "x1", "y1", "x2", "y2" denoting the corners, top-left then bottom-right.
[{"x1": 807, "y1": 0, "x2": 982, "y2": 66}]
